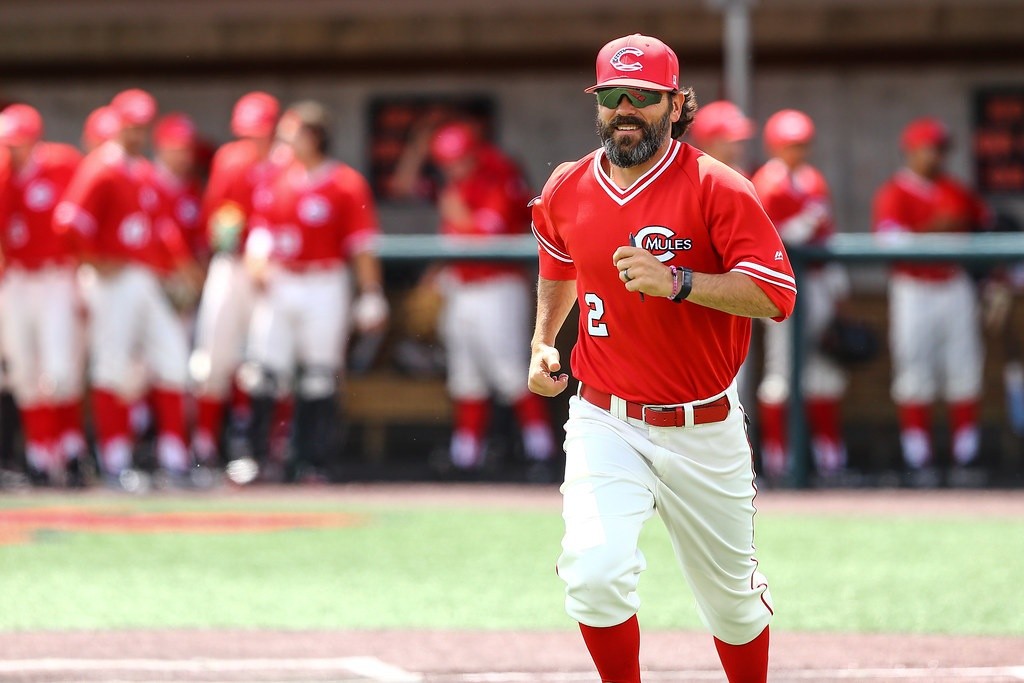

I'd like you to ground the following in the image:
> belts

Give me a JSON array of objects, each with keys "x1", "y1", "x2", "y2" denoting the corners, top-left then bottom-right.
[{"x1": 580, "y1": 383, "x2": 730, "y2": 427}]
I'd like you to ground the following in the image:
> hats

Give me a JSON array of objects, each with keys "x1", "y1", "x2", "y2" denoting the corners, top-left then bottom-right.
[
  {"x1": 584, "y1": 33, "x2": 680, "y2": 93},
  {"x1": 763, "y1": 111, "x2": 815, "y2": 147},
  {"x1": 88, "y1": 106, "x2": 127, "y2": 146},
  {"x1": 232, "y1": 92, "x2": 280, "y2": 136},
  {"x1": 154, "y1": 114, "x2": 195, "y2": 149},
  {"x1": 691, "y1": 101, "x2": 751, "y2": 142},
  {"x1": 432, "y1": 121, "x2": 482, "y2": 156},
  {"x1": 111, "y1": 88, "x2": 155, "y2": 126},
  {"x1": 903, "y1": 121, "x2": 949, "y2": 149},
  {"x1": 0, "y1": 103, "x2": 41, "y2": 146}
]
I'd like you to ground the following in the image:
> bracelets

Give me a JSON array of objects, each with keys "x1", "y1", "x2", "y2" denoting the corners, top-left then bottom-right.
[{"x1": 666, "y1": 265, "x2": 678, "y2": 301}]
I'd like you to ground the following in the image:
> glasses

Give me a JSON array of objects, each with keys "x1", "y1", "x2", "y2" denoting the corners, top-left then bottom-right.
[{"x1": 594, "y1": 88, "x2": 662, "y2": 110}]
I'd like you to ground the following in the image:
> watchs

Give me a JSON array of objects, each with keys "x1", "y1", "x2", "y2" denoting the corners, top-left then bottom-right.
[{"x1": 673, "y1": 266, "x2": 693, "y2": 304}]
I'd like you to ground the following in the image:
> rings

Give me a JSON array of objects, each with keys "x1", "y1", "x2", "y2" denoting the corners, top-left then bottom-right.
[{"x1": 623, "y1": 269, "x2": 631, "y2": 281}]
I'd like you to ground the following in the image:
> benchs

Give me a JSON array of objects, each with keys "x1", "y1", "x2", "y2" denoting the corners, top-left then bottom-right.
[{"x1": 338, "y1": 369, "x2": 460, "y2": 469}]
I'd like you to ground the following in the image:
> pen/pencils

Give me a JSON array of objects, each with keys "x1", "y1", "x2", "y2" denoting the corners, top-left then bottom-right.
[{"x1": 629, "y1": 233, "x2": 645, "y2": 302}]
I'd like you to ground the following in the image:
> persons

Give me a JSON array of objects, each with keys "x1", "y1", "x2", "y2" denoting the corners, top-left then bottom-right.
[
  {"x1": 527, "y1": 31, "x2": 797, "y2": 683},
  {"x1": 868, "y1": 116, "x2": 1015, "y2": 488},
  {"x1": 0, "y1": 89, "x2": 390, "y2": 496},
  {"x1": 691, "y1": 97, "x2": 864, "y2": 488},
  {"x1": 383, "y1": 107, "x2": 559, "y2": 484}
]
